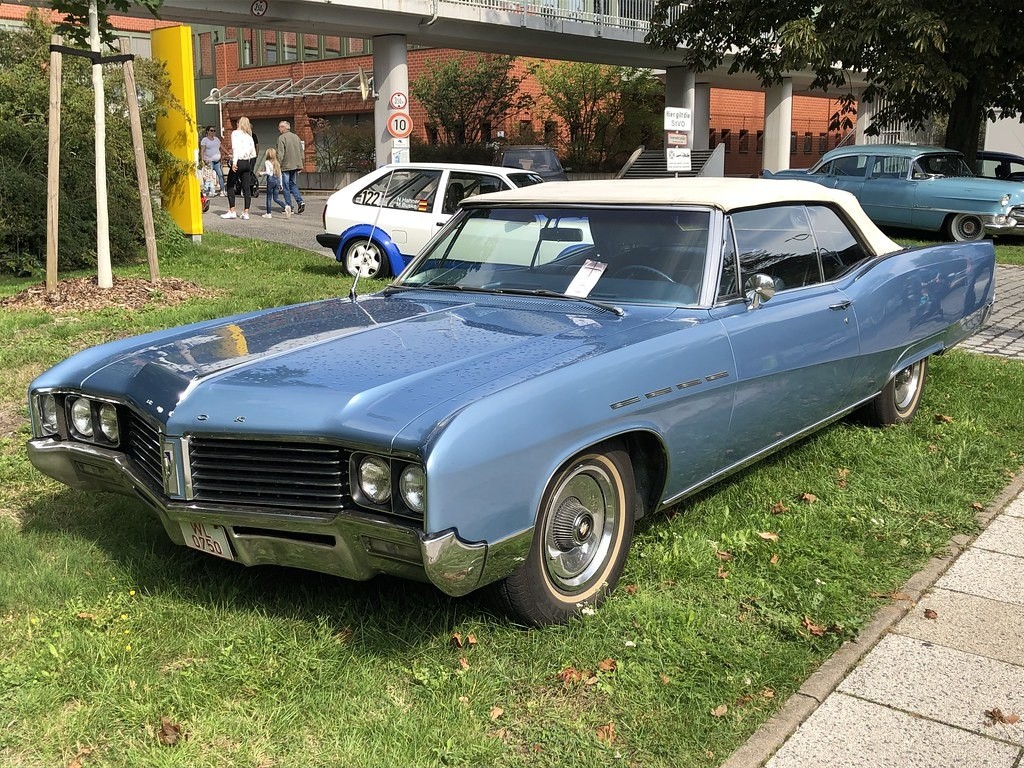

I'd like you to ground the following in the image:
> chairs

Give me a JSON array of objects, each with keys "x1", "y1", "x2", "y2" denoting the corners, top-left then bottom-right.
[
  {"x1": 446, "y1": 183, "x2": 463, "y2": 213},
  {"x1": 648, "y1": 243, "x2": 705, "y2": 294}
]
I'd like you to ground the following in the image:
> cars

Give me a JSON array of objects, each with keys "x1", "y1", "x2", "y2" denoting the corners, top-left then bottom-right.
[
  {"x1": 761, "y1": 143, "x2": 1024, "y2": 243},
  {"x1": 487, "y1": 143, "x2": 573, "y2": 183},
  {"x1": 316, "y1": 162, "x2": 594, "y2": 284}
]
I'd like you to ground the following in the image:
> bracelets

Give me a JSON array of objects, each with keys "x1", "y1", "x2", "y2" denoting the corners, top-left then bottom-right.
[{"x1": 227, "y1": 153, "x2": 231, "y2": 156}]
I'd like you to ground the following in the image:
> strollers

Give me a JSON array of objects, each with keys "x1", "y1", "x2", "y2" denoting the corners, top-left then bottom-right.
[{"x1": 226, "y1": 157, "x2": 260, "y2": 198}]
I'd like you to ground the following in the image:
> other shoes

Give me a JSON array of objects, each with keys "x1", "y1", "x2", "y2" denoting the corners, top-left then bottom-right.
[
  {"x1": 202, "y1": 199, "x2": 210, "y2": 212},
  {"x1": 220, "y1": 194, "x2": 228, "y2": 196},
  {"x1": 282, "y1": 209, "x2": 294, "y2": 214},
  {"x1": 298, "y1": 202, "x2": 305, "y2": 213}
]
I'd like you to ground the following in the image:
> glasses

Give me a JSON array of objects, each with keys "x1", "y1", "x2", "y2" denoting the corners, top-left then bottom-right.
[{"x1": 209, "y1": 130, "x2": 216, "y2": 132}]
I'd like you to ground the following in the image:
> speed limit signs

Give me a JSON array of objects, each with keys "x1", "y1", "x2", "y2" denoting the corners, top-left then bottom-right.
[{"x1": 387, "y1": 112, "x2": 414, "y2": 138}]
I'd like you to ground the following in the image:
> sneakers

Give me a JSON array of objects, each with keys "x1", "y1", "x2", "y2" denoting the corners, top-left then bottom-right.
[
  {"x1": 285, "y1": 205, "x2": 291, "y2": 218},
  {"x1": 220, "y1": 211, "x2": 237, "y2": 218},
  {"x1": 240, "y1": 213, "x2": 249, "y2": 220},
  {"x1": 262, "y1": 213, "x2": 272, "y2": 218}
]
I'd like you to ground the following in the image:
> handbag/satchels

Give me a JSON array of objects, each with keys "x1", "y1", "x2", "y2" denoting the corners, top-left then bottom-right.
[{"x1": 241, "y1": 171, "x2": 259, "y2": 198}]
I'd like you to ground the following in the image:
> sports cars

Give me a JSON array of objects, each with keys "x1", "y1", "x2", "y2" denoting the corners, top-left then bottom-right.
[{"x1": 25, "y1": 177, "x2": 997, "y2": 632}]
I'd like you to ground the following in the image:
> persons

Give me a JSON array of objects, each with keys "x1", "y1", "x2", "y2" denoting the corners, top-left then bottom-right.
[
  {"x1": 201, "y1": 125, "x2": 232, "y2": 196},
  {"x1": 276, "y1": 121, "x2": 306, "y2": 214},
  {"x1": 221, "y1": 117, "x2": 259, "y2": 219},
  {"x1": 257, "y1": 148, "x2": 291, "y2": 220}
]
